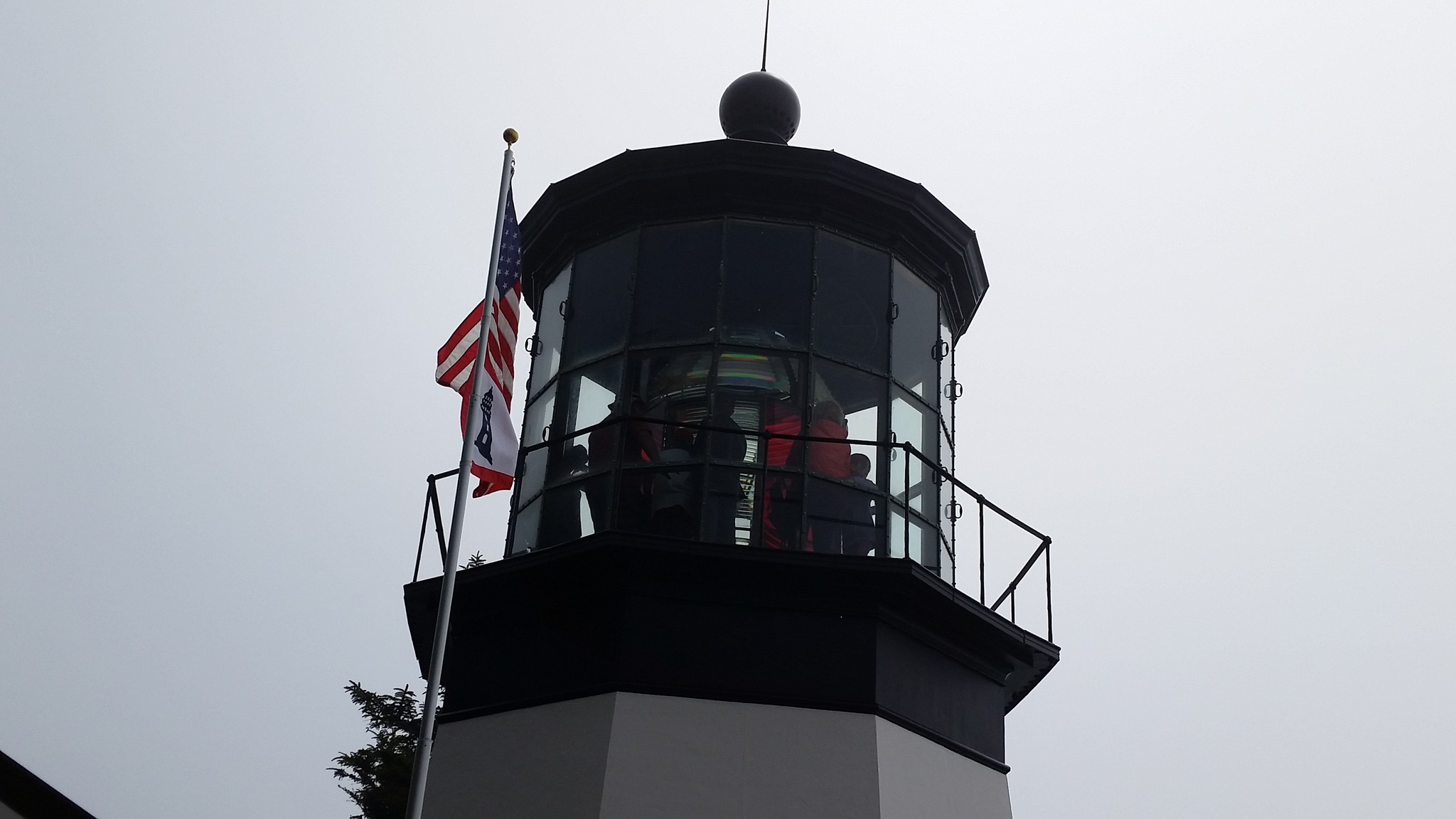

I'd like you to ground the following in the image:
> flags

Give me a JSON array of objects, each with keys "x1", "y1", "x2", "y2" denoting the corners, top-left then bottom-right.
[{"x1": 434, "y1": 170, "x2": 523, "y2": 498}]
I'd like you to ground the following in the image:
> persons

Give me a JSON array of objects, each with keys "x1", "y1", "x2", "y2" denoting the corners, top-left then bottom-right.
[{"x1": 553, "y1": 385, "x2": 880, "y2": 555}]
